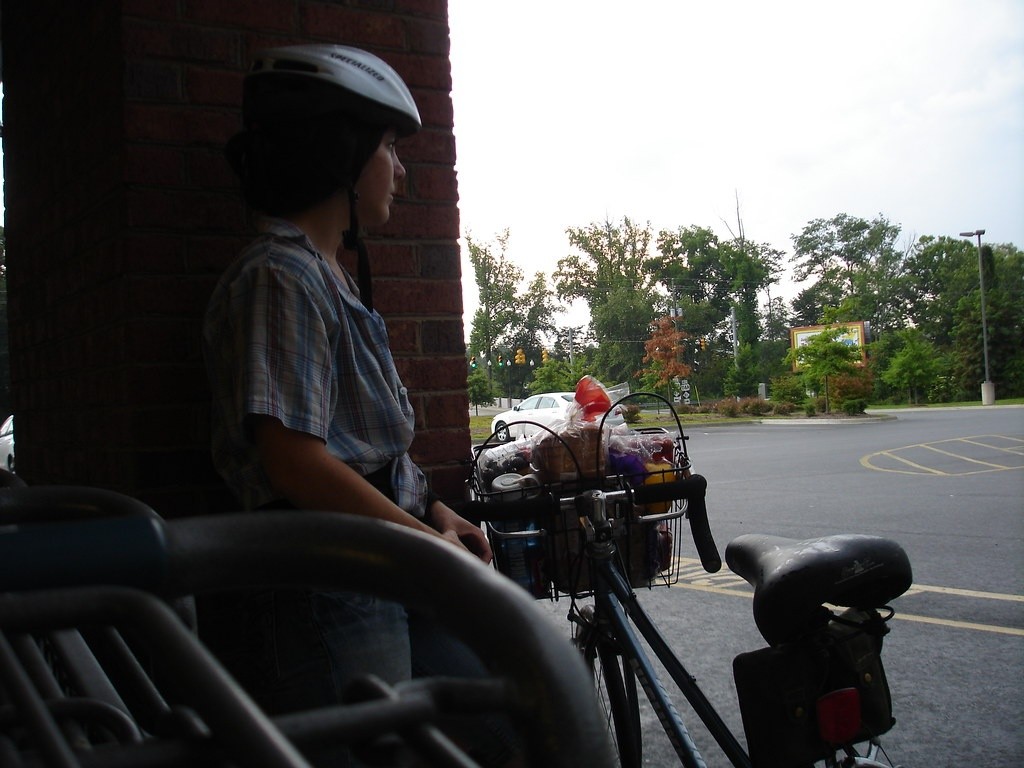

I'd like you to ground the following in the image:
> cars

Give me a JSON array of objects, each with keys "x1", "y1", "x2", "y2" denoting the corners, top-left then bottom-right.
[{"x1": 490, "y1": 392, "x2": 625, "y2": 443}]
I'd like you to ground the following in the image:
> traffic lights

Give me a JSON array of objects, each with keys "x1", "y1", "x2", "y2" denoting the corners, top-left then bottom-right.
[
  {"x1": 498, "y1": 356, "x2": 503, "y2": 368},
  {"x1": 700, "y1": 339, "x2": 705, "y2": 351},
  {"x1": 471, "y1": 355, "x2": 476, "y2": 369},
  {"x1": 521, "y1": 353, "x2": 525, "y2": 364},
  {"x1": 541, "y1": 350, "x2": 549, "y2": 362},
  {"x1": 514, "y1": 349, "x2": 521, "y2": 364}
]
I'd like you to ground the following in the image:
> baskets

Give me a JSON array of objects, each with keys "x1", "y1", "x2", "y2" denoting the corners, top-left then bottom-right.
[{"x1": 470, "y1": 428, "x2": 690, "y2": 602}]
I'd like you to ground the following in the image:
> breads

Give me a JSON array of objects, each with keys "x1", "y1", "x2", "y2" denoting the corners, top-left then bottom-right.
[{"x1": 539, "y1": 426, "x2": 605, "y2": 482}]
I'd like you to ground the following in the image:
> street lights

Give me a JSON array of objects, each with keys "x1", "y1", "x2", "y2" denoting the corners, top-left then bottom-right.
[{"x1": 958, "y1": 229, "x2": 992, "y2": 383}]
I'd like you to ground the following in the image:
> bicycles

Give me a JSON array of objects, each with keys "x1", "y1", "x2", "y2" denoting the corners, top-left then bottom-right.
[{"x1": 469, "y1": 390, "x2": 916, "y2": 768}]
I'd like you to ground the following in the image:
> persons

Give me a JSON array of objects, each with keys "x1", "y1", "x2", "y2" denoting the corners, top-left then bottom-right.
[{"x1": 205, "y1": 42, "x2": 524, "y2": 768}]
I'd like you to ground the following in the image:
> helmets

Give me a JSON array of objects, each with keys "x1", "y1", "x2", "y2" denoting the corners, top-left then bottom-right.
[{"x1": 242, "y1": 44, "x2": 422, "y2": 138}]
